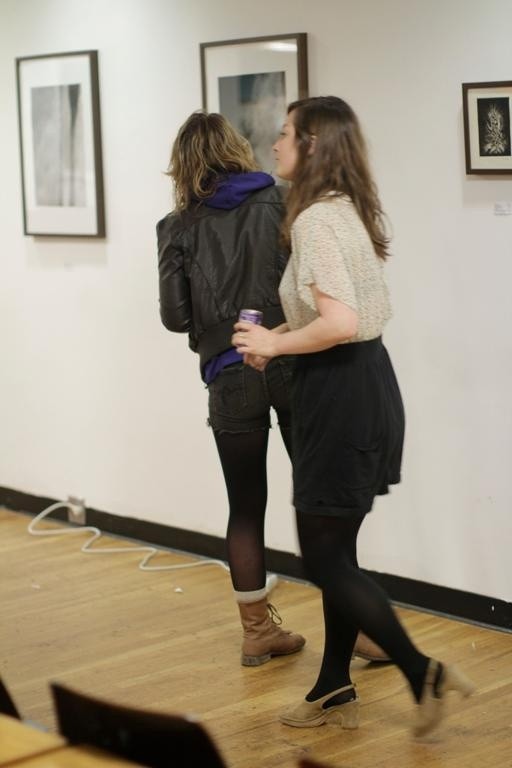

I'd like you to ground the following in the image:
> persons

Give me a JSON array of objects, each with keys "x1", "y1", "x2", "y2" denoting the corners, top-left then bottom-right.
[
  {"x1": 232, "y1": 95, "x2": 482, "y2": 739},
  {"x1": 152, "y1": 105, "x2": 416, "y2": 669}
]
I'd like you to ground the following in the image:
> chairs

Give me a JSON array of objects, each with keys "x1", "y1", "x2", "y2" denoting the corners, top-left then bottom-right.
[{"x1": 47, "y1": 678, "x2": 223, "y2": 767}]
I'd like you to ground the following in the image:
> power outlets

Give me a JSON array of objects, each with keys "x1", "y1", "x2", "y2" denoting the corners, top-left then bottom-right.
[{"x1": 67, "y1": 496, "x2": 86, "y2": 526}]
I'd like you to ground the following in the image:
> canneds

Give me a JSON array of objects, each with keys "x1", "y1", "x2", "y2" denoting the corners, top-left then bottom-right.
[{"x1": 236, "y1": 309, "x2": 264, "y2": 348}]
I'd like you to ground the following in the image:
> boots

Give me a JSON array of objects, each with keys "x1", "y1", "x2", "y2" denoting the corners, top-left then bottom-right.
[
  {"x1": 236, "y1": 586, "x2": 308, "y2": 669},
  {"x1": 350, "y1": 628, "x2": 393, "y2": 666}
]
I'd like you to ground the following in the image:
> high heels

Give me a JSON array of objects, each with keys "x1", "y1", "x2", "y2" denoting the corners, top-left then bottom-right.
[
  {"x1": 275, "y1": 681, "x2": 364, "y2": 734},
  {"x1": 411, "y1": 662, "x2": 479, "y2": 740}
]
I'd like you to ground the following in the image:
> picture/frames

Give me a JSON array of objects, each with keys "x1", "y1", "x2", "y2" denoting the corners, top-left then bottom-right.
[
  {"x1": 462, "y1": 81, "x2": 512, "y2": 174},
  {"x1": 199, "y1": 32, "x2": 307, "y2": 190},
  {"x1": 15, "y1": 49, "x2": 104, "y2": 237}
]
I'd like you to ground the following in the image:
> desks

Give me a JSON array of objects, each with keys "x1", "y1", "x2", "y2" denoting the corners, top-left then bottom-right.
[{"x1": 1, "y1": 714, "x2": 133, "y2": 766}]
